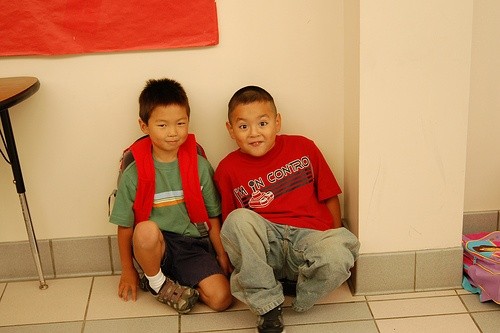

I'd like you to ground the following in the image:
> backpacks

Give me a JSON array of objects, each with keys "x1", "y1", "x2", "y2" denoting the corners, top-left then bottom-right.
[
  {"x1": 460, "y1": 228, "x2": 500, "y2": 306},
  {"x1": 119, "y1": 135, "x2": 212, "y2": 237}
]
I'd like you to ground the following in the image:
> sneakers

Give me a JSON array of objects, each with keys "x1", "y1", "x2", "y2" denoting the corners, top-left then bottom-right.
[
  {"x1": 255, "y1": 303, "x2": 285, "y2": 333},
  {"x1": 278, "y1": 280, "x2": 298, "y2": 298},
  {"x1": 145, "y1": 277, "x2": 198, "y2": 313}
]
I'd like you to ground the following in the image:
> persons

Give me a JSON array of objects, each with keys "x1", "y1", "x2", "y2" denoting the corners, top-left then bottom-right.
[
  {"x1": 209, "y1": 85, "x2": 361, "y2": 333},
  {"x1": 106, "y1": 79, "x2": 232, "y2": 315}
]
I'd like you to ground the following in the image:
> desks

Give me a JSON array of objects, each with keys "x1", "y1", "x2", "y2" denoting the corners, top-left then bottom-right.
[{"x1": 0, "y1": 76, "x2": 49, "y2": 291}]
